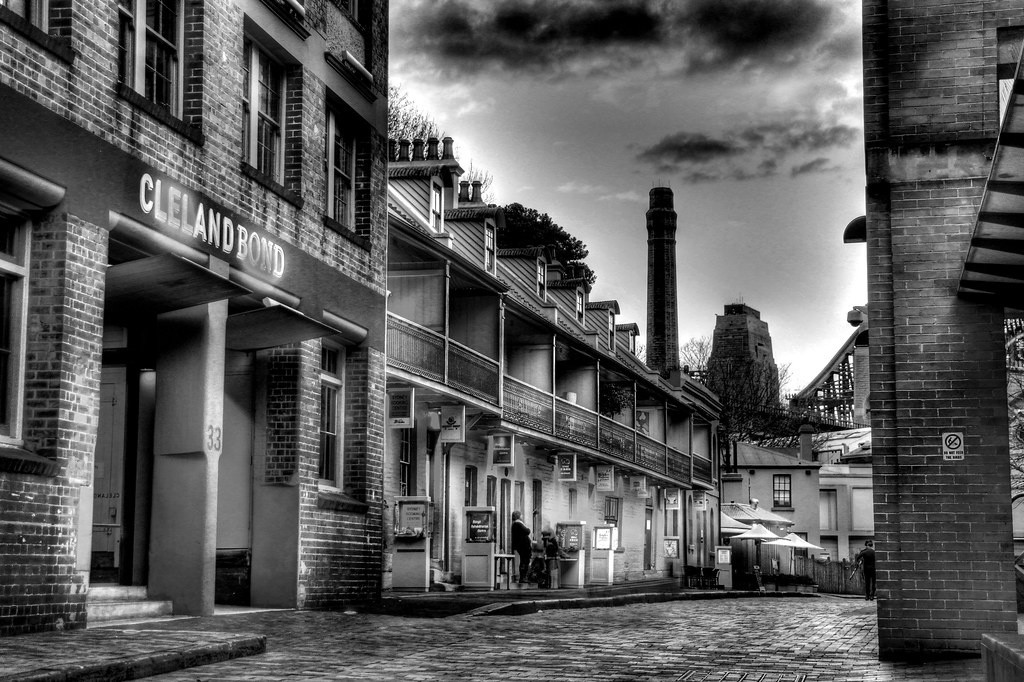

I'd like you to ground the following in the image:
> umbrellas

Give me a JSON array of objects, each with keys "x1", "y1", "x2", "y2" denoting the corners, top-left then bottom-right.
[
  {"x1": 729, "y1": 524, "x2": 792, "y2": 568},
  {"x1": 720, "y1": 511, "x2": 752, "y2": 547},
  {"x1": 762, "y1": 533, "x2": 825, "y2": 575}
]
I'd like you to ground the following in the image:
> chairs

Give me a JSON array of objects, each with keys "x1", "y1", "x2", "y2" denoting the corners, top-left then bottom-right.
[{"x1": 683, "y1": 565, "x2": 720, "y2": 590}]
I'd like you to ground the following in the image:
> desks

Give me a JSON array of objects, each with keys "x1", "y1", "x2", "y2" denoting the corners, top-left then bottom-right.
[{"x1": 494, "y1": 554, "x2": 515, "y2": 590}]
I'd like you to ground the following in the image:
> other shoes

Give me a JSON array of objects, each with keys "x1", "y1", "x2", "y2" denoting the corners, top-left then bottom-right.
[
  {"x1": 870, "y1": 596, "x2": 874, "y2": 600},
  {"x1": 865, "y1": 596, "x2": 869, "y2": 601},
  {"x1": 519, "y1": 577, "x2": 528, "y2": 583}
]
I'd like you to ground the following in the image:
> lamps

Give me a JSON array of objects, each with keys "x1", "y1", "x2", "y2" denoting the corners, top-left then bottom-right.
[{"x1": 847, "y1": 303, "x2": 868, "y2": 327}]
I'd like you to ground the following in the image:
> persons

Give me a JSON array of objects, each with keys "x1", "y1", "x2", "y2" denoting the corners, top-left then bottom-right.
[
  {"x1": 855, "y1": 540, "x2": 876, "y2": 601},
  {"x1": 543, "y1": 536, "x2": 561, "y2": 589},
  {"x1": 512, "y1": 511, "x2": 533, "y2": 584}
]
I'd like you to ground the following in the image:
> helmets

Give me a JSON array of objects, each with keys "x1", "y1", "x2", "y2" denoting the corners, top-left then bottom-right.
[{"x1": 865, "y1": 540, "x2": 873, "y2": 547}]
